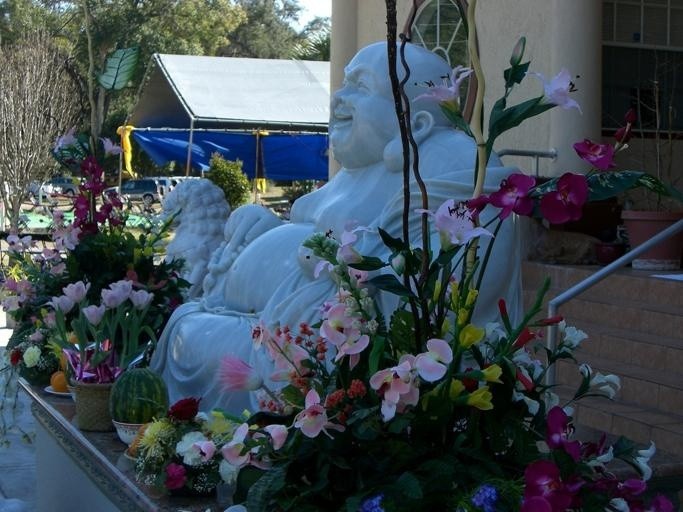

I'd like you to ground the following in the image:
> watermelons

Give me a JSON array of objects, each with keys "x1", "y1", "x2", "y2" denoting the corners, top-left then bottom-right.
[{"x1": 109, "y1": 369, "x2": 169, "y2": 424}]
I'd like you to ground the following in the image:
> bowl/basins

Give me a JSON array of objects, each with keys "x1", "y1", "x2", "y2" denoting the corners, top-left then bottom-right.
[{"x1": 112, "y1": 419, "x2": 144, "y2": 444}]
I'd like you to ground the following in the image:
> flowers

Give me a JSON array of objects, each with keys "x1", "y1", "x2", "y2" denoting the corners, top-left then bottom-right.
[
  {"x1": 31, "y1": 279, "x2": 166, "y2": 384},
  {"x1": 136, "y1": 396, "x2": 236, "y2": 494},
  {"x1": 1, "y1": 124, "x2": 192, "y2": 354}
]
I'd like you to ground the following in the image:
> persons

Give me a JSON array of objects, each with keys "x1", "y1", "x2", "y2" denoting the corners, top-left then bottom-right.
[{"x1": 146, "y1": 40, "x2": 523, "y2": 417}]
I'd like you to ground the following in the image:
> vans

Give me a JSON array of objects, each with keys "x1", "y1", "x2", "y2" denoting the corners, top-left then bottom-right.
[{"x1": 46, "y1": 178, "x2": 87, "y2": 197}]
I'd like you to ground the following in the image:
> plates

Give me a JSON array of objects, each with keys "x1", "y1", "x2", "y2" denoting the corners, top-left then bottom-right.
[{"x1": 44, "y1": 384, "x2": 70, "y2": 399}]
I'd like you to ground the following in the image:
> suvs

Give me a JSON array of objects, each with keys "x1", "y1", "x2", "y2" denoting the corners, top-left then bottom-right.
[{"x1": 102, "y1": 179, "x2": 165, "y2": 208}]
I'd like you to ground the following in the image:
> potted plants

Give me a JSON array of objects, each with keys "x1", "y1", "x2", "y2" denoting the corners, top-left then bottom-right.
[{"x1": 621, "y1": 174, "x2": 683, "y2": 274}]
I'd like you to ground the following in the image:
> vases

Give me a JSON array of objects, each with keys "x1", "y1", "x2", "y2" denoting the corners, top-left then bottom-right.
[{"x1": 66, "y1": 382, "x2": 118, "y2": 433}]
